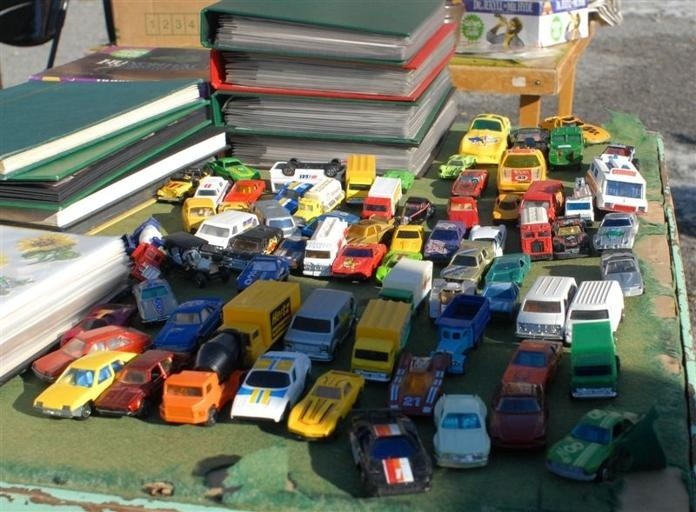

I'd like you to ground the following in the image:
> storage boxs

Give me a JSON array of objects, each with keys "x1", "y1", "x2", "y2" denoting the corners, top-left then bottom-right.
[{"x1": 112, "y1": 0, "x2": 214, "y2": 49}]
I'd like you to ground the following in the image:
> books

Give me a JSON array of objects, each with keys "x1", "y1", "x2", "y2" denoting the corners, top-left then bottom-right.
[
  {"x1": 0, "y1": 1, "x2": 461, "y2": 245},
  {"x1": 0, "y1": 227, "x2": 135, "y2": 392}
]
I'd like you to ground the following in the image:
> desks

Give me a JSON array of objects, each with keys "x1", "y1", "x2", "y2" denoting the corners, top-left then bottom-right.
[{"x1": 451, "y1": 18, "x2": 593, "y2": 125}]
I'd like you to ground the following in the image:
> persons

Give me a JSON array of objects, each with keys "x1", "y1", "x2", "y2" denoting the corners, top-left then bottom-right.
[
  {"x1": 486, "y1": 17, "x2": 526, "y2": 50},
  {"x1": 565, "y1": 13, "x2": 581, "y2": 43}
]
